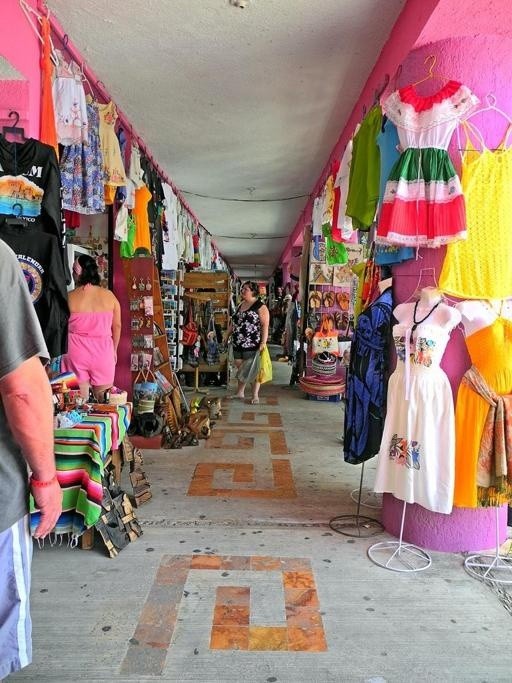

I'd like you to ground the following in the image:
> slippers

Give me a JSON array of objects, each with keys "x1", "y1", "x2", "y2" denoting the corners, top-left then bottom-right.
[
  {"x1": 226, "y1": 394, "x2": 245, "y2": 399},
  {"x1": 249, "y1": 397, "x2": 261, "y2": 405},
  {"x1": 322, "y1": 290, "x2": 330, "y2": 307},
  {"x1": 315, "y1": 289, "x2": 322, "y2": 308},
  {"x1": 337, "y1": 292, "x2": 347, "y2": 310},
  {"x1": 309, "y1": 310, "x2": 352, "y2": 332},
  {"x1": 343, "y1": 291, "x2": 350, "y2": 310},
  {"x1": 309, "y1": 289, "x2": 316, "y2": 308},
  {"x1": 328, "y1": 290, "x2": 336, "y2": 306}
]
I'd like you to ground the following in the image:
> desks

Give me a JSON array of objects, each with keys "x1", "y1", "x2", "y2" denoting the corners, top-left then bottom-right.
[{"x1": 25, "y1": 400, "x2": 137, "y2": 553}]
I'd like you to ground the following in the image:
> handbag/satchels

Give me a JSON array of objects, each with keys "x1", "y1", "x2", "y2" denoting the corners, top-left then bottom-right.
[
  {"x1": 131, "y1": 367, "x2": 159, "y2": 414},
  {"x1": 180, "y1": 299, "x2": 224, "y2": 368},
  {"x1": 311, "y1": 330, "x2": 339, "y2": 359},
  {"x1": 310, "y1": 351, "x2": 338, "y2": 379}
]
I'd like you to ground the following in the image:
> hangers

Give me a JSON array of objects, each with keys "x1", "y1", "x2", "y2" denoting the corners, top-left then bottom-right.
[
  {"x1": 453, "y1": 95, "x2": 512, "y2": 151},
  {"x1": 0, "y1": 107, "x2": 29, "y2": 143},
  {"x1": 3, "y1": 203, "x2": 29, "y2": 229},
  {"x1": 60, "y1": 32, "x2": 110, "y2": 103},
  {"x1": 408, "y1": 52, "x2": 452, "y2": 87}
]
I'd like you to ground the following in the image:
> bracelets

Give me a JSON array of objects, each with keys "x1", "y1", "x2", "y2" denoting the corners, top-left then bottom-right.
[{"x1": 30, "y1": 475, "x2": 57, "y2": 488}]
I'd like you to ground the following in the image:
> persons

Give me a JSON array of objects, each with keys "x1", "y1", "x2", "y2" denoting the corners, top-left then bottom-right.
[
  {"x1": 372, "y1": 287, "x2": 462, "y2": 515},
  {"x1": 224, "y1": 282, "x2": 270, "y2": 404},
  {"x1": 344, "y1": 278, "x2": 393, "y2": 465},
  {"x1": 0, "y1": 238, "x2": 64, "y2": 683},
  {"x1": 60, "y1": 254, "x2": 121, "y2": 401},
  {"x1": 453, "y1": 300, "x2": 512, "y2": 509}
]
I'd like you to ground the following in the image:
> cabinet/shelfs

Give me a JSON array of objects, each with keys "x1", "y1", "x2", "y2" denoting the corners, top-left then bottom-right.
[{"x1": 129, "y1": 254, "x2": 174, "y2": 397}]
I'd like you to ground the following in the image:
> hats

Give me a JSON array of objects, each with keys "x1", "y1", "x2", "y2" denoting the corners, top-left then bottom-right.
[
  {"x1": 282, "y1": 293, "x2": 293, "y2": 302},
  {"x1": 318, "y1": 351, "x2": 332, "y2": 364}
]
